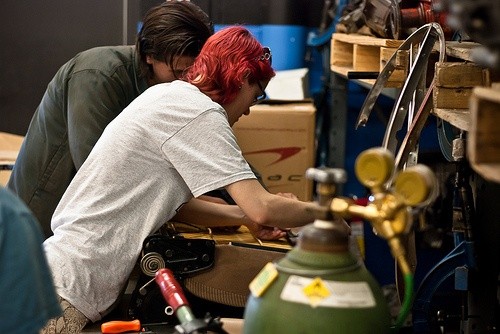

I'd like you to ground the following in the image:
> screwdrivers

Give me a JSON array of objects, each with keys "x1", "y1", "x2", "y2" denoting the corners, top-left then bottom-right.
[{"x1": 101, "y1": 319, "x2": 169, "y2": 334}]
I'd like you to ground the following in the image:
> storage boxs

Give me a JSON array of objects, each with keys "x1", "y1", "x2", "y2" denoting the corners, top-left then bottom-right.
[{"x1": 231, "y1": 103, "x2": 314, "y2": 202}]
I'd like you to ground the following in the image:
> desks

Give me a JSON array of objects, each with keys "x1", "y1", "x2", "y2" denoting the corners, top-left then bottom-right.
[{"x1": 0, "y1": 130, "x2": 327, "y2": 251}]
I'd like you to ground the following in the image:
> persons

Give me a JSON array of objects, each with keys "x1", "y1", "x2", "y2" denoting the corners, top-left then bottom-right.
[
  {"x1": 6, "y1": 0, "x2": 298, "y2": 239},
  {"x1": 39, "y1": 26, "x2": 328, "y2": 334},
  {"x1": 0, "y1": 185, "x2": 62, "y2": 334}
]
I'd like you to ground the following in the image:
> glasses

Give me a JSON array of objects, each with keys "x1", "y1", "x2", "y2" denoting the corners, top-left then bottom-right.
[{"x1": 258, "y1": 47, "x2": 272, "y2": 67}]
